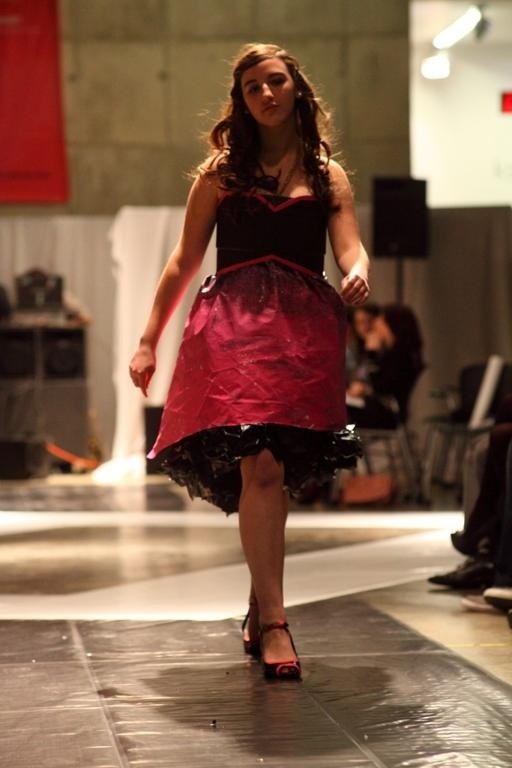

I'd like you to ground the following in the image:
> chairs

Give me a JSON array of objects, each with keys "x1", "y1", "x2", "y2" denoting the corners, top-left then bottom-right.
[{"x1": 350, "y1": 355, "x2": 495, "y2": 507}]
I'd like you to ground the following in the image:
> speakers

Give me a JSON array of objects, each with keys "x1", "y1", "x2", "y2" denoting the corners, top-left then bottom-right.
[{"x1": 369, "y1": 176, "x2": 429, "y2": 258}]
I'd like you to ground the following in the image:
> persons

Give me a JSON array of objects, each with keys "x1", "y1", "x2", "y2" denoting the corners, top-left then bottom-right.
[
  {"x1": 428, "y1": 394, "x2": 512, "y2": 610},
  {"x1": 129, "y1": 42, "x2": 371, "y2": 681},
  {"x1": 344, "y1": 302, "x2": 427, "y2": 427}
]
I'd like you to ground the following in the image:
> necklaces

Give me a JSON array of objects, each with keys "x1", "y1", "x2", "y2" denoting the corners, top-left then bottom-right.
[{"x1": 257, "y1": 146, "x2": 301, "y2": 196}]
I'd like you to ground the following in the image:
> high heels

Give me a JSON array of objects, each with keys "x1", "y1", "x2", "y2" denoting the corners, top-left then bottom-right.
[{"x1": 241, "y1": 606, "x2": 305, "y2": 682}]
